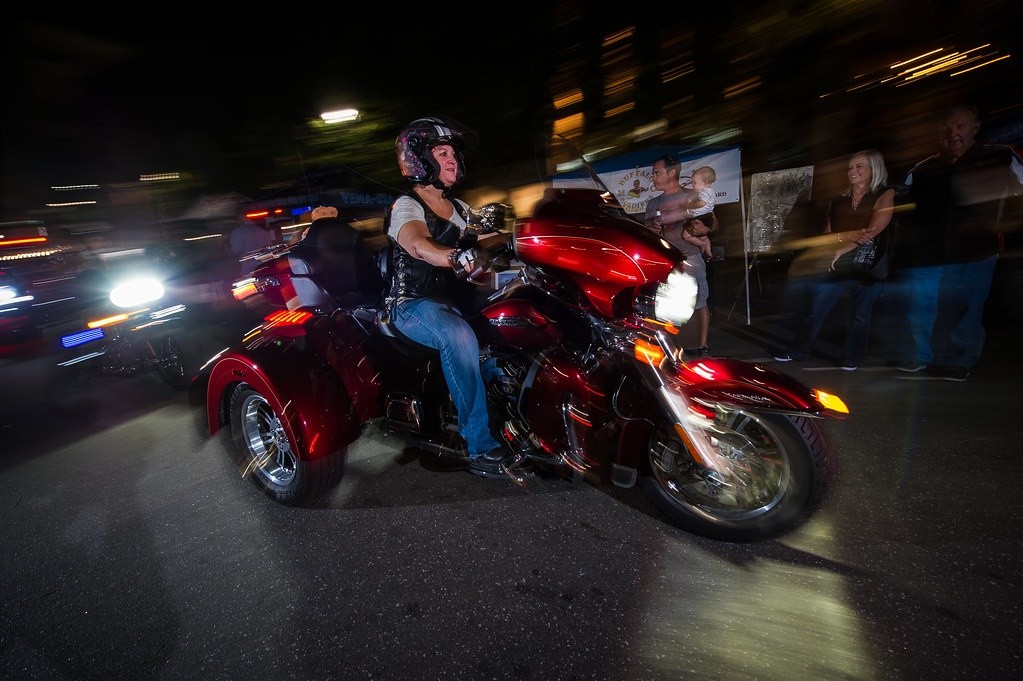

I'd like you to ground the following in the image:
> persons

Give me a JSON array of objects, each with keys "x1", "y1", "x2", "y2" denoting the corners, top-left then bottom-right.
[
  {"x1": 228, "y1": 205, "x2": 276, "y2": 289},
  {"x1": 896, "y1": 104, "x2": 1023, "y2": 382},
  {"x1": 384, "y1": 118, "x2": 515, "y2": 479},
  {"x1": 774, "y1": 148, "x2": 895, "y2": 371},
  {"x1": 644, "y1": 155, "x2": 719, "y2": 355}
]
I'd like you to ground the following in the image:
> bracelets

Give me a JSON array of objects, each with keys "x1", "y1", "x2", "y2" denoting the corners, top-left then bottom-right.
[{"x1": 837, "y1": 232, "x2": 843, "y2": 243}]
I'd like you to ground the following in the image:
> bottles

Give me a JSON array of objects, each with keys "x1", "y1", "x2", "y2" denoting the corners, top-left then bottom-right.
[{"x1": 656, "y1": 208, "x2": 665, "y2": 237}]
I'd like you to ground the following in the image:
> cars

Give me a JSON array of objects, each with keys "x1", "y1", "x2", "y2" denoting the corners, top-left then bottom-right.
[{"x1": 0, "y1": 221, "x2": 203, "y2": 436}]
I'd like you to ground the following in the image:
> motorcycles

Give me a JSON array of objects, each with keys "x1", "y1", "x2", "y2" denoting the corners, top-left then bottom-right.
[{"x1": 191, "y1": 132, "x2": 851, "y2": 546}]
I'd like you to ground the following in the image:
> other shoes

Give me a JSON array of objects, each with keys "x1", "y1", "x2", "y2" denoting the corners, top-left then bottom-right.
[
  {"x1": 698, "y1": 345, "x2": 711, "y2": 359},
  {"x1": 898, "y1": 359, "x2": 927, "y2": 372},
  {"x1": 839, "y1": 359, "x2": 858, "y2": 371},
  {"x1": 940, "y1": 369, "x2": 967, "y2": 381},
  {"x1": 775, "y1": 353, "x2": 794, "y2": 361}
]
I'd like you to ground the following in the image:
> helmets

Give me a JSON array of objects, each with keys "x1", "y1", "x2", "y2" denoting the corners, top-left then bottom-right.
[{"x1": 396, "y1": 116, "x2": 464, "y2": 182}]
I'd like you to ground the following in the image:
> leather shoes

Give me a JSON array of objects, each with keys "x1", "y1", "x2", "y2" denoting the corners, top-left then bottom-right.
[{"x1": 476, "y1": 446, "x2": 509, "y2": 467}]
